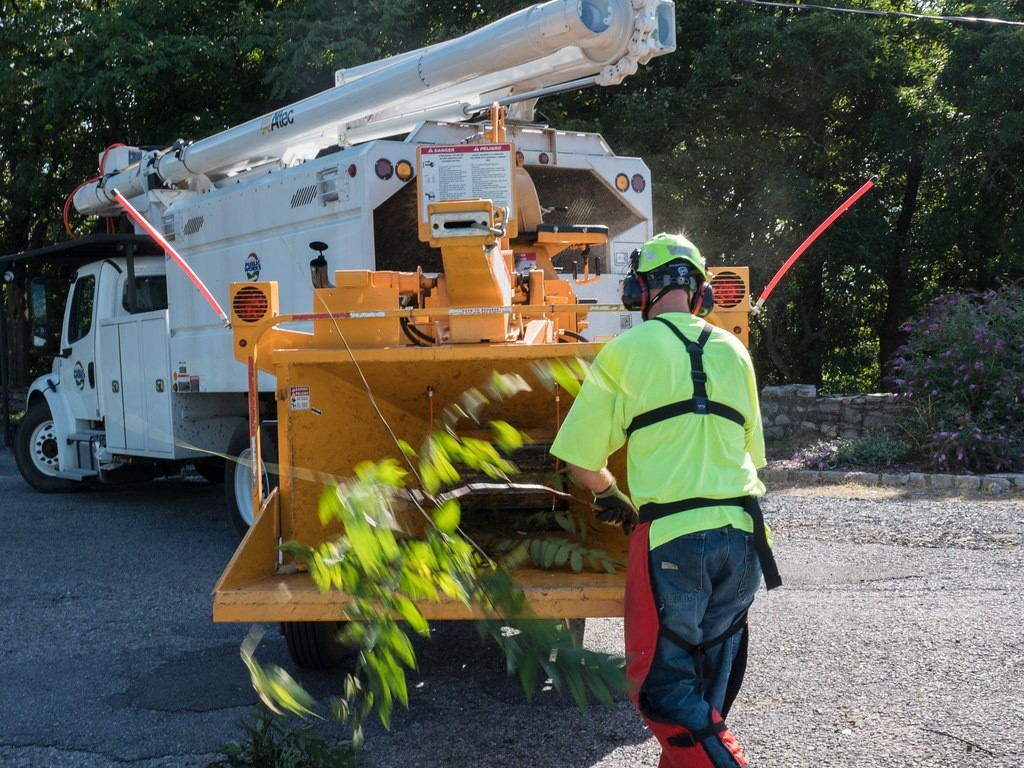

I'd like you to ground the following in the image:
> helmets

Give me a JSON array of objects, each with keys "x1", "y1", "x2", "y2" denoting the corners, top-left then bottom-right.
[{"x1": 636, "y1": 232, "x2": 707, "y2": 271}]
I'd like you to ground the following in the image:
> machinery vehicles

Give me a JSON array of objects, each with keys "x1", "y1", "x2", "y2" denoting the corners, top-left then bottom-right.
[
  {"x1": 14, "y1": 0, "x2": 753, "y2": 541},
  {"x1": 212, "y1": 105, "x2": 751, "y2": 664}
]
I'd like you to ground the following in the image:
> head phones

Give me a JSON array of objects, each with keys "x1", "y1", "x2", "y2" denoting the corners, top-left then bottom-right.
[{"x1": 622, "y1": 248, "x2": 714, "y2": 318}]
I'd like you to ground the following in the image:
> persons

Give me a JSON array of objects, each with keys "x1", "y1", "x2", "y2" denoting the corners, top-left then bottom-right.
[{"x1": 549, "y1": 233, "x2": 772, "y2": 768}]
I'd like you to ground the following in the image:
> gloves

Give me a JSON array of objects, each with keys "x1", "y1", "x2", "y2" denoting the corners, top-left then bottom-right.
[{"x1": 592, "y1": 476, "x2": 639, "y2": 535}]
